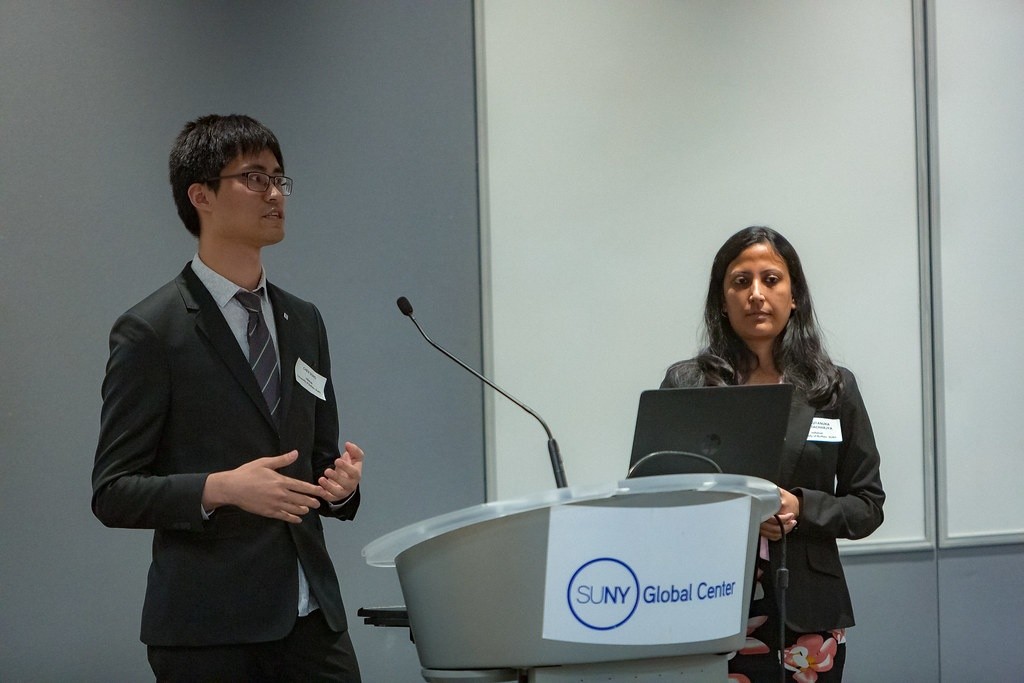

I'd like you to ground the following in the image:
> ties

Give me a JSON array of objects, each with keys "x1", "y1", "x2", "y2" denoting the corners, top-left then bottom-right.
[{"x1": 234, "y1": 290, "x2": 281, "y2": 438}]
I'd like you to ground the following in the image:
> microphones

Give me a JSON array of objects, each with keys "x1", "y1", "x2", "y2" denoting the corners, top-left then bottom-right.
[{"x1": 397, "y1": 296, "x2": 568, "y2": 489}]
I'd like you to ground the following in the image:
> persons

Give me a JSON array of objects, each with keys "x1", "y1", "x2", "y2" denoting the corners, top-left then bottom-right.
[
  {"x1": 659, "y1": 226, "x2": 886, "y2": 683},
  {"x1": 91, "y1": 113, "x2": 365, "y2": 682}
]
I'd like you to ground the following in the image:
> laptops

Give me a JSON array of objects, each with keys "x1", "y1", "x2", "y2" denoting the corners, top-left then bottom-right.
[{"x1": 629, "y1": 383, "x2": 794, "y2": 487}]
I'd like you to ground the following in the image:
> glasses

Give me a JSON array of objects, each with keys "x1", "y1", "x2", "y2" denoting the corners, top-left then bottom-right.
[{"x1": 195, "y1": 170, "x2": 293, "y2": 197}]
